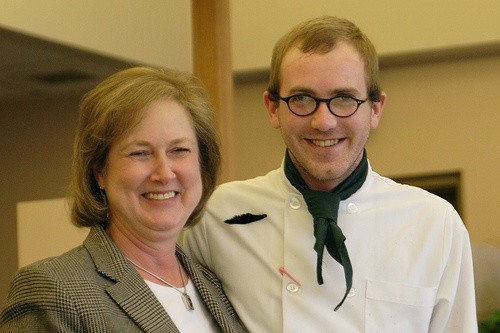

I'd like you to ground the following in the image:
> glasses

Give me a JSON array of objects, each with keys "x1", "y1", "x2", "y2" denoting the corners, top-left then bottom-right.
[{"x1": 271, "y1": 89, "x2": 372, "y2": 118}]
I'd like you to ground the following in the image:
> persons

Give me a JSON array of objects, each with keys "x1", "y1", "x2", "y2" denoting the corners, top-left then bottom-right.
[
  {"x1": 173, "y1": 15, "x2": 477, "y2": 332},
  {"x1": 0, "y1": 67, "x2": 248, "y2": 333}
]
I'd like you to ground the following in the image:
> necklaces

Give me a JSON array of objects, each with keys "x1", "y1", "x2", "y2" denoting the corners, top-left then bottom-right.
[{"x1": 122, "y1": 254, "x2": 195, "y2": 312}]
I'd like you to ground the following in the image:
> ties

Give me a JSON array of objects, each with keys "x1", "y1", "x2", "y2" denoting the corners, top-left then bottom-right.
[{"x1": 283, "y1": 144, "x2": 368, "y2": 312}]
